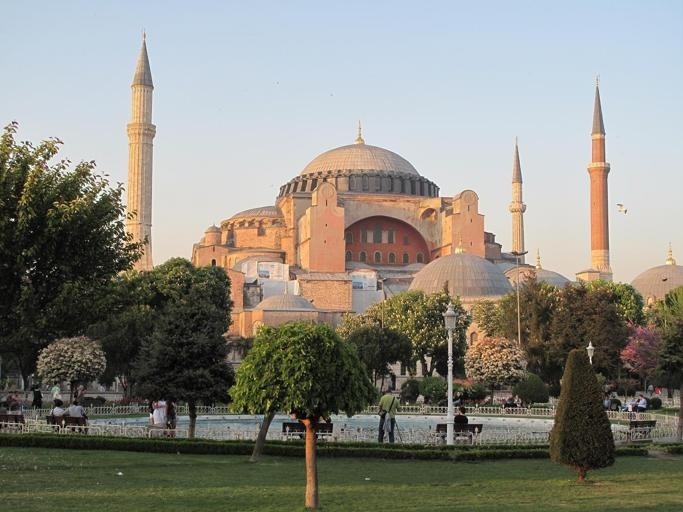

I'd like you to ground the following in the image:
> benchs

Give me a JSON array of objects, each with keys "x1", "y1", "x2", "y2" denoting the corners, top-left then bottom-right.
[
  {"x1": 614, "y1": 421, "x2": 656, "y2": 444},
  {"x1": 0, "y1": 415, "x2": 25, "y2": 430},
  {"x1": 80, "y1": 397, "x2": 103, "y2": 407},
  {"x1": 281, "y1": 422, "x2": 333, "y2": 441},
  {"x1": 45, "y1": 416, "x2": 89, "y2": 434},
  {"x1": 432, "y1": 424, "x2": 482, "y2": 445}
]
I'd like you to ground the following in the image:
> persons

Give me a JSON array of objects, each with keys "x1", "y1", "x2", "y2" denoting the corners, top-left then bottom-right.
[
  {"x1": 62, "y1": 400, "x2": 88, "y2": 434},
  {"x1": 50, "y1": 381, "x2": 61, "y2": 403},
  {"x1": 6, "y1": 391, "x2": 20, "y2": 410},
  {"x1": 503, "y1": 394, "x2": 513, "y2": 407},
  {"x1": 601, "y1": 383, "x2": 662, "y2": 412},
  {"x1": 511, "y1": 395, "x2": 521, "y2": 409},
  {"x1": 378, "y1": 389, "x2": 401, "y2": 442},
  {"x1": 148, "y1": 400, "x2": 177, "y2": 437},
  {"x1": 50, "y1": 399, "x2": 65, "y2": 432},
  {"x1": 31, "y1": 384, "x2": 42, "y2": 409},
  {"x1": 12, "y1": 391, "x2": 28, "y2": 410},
  {"x1": 454, "y1": 406, "x2": 467, "y2": 436}
]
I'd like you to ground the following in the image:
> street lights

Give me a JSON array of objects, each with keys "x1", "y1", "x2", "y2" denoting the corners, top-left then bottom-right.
[
  {"x1": 506, "y1": 248, "x2": 531, "y2": 347},
  {"x1": 586, "y1": 342, "x2": 594, "y2": 365},
  {"x1": 439, "y1": 301, "x2": 457, "y2": 445},
  {"x1": 378, "y1": 276, "x2": 389, "y2": 331}
]
